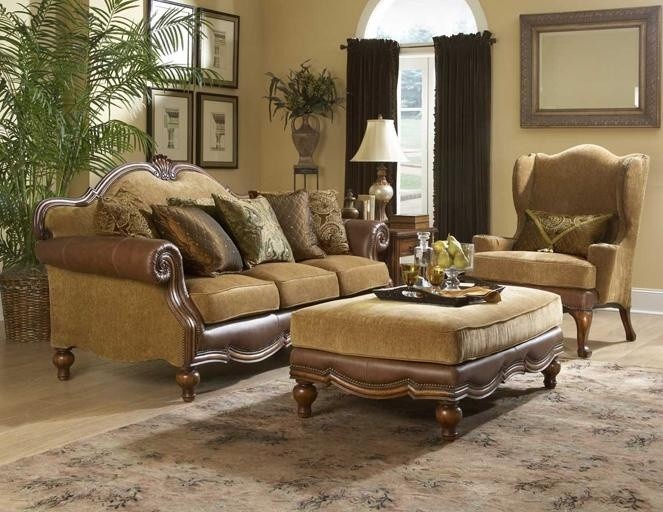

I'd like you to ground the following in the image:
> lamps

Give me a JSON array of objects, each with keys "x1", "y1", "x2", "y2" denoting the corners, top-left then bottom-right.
[{"x1": 348, "y1": 113, "x2": 410, "y2": 230}]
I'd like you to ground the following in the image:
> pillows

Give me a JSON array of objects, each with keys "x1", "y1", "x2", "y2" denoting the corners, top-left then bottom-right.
[
  {"x1": 211, "y1": 187, "x2": 292, "y2": 267},
  {"x1": 245, "y1": 183, "x2": 325, "y2": 260},
  {"x1": 153, "y1": 199, "x2": 242, "y2": 277},
  {"x1": 510, "y1": 206, "x2": 613, "y2": 259},
  {"x1": 89, "y1": 184, "x2": 156, "y2": 239},
  {"x1": 300, "y1": 185, "x2": 349, "y2": 253},
  {"x1": 166, "y1": 196, "x2": 216, "y2": 206}
]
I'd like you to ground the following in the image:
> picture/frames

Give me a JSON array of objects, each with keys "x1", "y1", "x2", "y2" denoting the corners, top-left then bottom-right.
[
  {"x1": 138, "y1": 0, "x2": 197, "y2": 86},
  {"x1": 516, "y1": 2, "x2": 661, "y2": 129},
  {"x1": 145, "y1": 85, "x2": 196, "y2": 170},
  {"x1": 195, "y1": 91, "x2": 239, "y2": 170},
  {"x1": 192, "y1": 4, "x2": 241, "y2": 90}
]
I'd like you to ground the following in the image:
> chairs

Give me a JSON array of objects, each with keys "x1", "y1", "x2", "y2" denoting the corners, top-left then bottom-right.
[{"x1": 460, "y1": 142, "x2": 650, "y2": 359}]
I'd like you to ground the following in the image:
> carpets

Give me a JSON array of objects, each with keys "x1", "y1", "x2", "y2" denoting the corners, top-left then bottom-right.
[{"x1": 0, "y1": 352, "x2": 660, "y2": 512}]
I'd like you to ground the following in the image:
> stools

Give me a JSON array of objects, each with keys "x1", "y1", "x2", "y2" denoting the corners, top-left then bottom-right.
[{"x1": 286, "y1": 281, "x2": 568, "y2": 442}]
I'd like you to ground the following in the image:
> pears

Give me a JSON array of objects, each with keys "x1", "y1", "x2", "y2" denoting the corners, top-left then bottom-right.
[{"x1": 433, "y1": 232, "x2": 469, "y2": 269}]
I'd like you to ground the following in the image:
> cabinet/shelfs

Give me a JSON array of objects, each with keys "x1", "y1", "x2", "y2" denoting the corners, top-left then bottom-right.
[{"x1": 290, "y1": 166, "x2": 322, "y2": 194}]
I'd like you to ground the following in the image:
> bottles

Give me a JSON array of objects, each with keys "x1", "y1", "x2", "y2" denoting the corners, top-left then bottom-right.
[
  {"x1": 340, "y1": 191, "x2": 359, "y2": 219},
  {"x1": 413, "y1": 232, "x2": 433, "y2": 278}
]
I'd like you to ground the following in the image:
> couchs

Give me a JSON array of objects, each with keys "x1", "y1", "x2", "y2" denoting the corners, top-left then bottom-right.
[{"x1": 34, "y1": 150, "x2": 395, "y2": 405}]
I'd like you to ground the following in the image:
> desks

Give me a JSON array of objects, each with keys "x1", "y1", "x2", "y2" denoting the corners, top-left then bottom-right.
[{"x1": 378, "y1": 227, "x2": 440, "y2": 284}]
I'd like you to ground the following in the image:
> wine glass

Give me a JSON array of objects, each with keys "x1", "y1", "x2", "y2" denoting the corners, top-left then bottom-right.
[
  {"x1": 399, "y1": 263, "x2": 419, "y2": 297},
  {"x1": 426, "y1": 242, "x2": 474, "y2": 296}
]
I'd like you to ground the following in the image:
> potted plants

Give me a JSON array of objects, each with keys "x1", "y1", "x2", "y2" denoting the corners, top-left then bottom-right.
[
  {"x1": 0, "y1": 0, "x2": 227, "y2": 346},
  {"x1": 261, "y1": 58, "x2": 346, "y2": 168}
]
